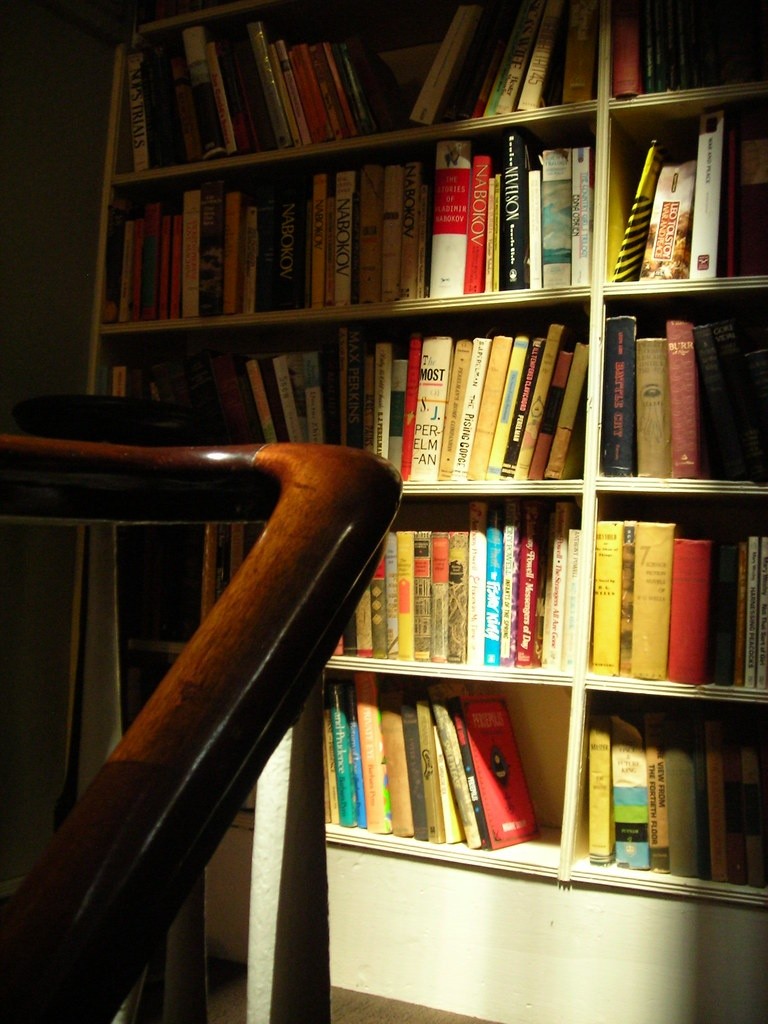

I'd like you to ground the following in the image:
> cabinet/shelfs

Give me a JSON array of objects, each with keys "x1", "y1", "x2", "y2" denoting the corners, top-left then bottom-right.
[{"x1": 53, "y1": 0, "x2": 768, "y2": 1024}]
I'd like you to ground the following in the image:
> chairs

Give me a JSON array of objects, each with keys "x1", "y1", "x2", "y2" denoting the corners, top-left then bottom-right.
[{"x1": 0, "y1": 431, "x2": 399, "y2": 1024}]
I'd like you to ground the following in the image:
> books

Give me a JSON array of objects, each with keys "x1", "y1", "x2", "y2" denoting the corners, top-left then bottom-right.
[{"x1": 119, "y1": 0, "x2": 768, "y2": 890}]
